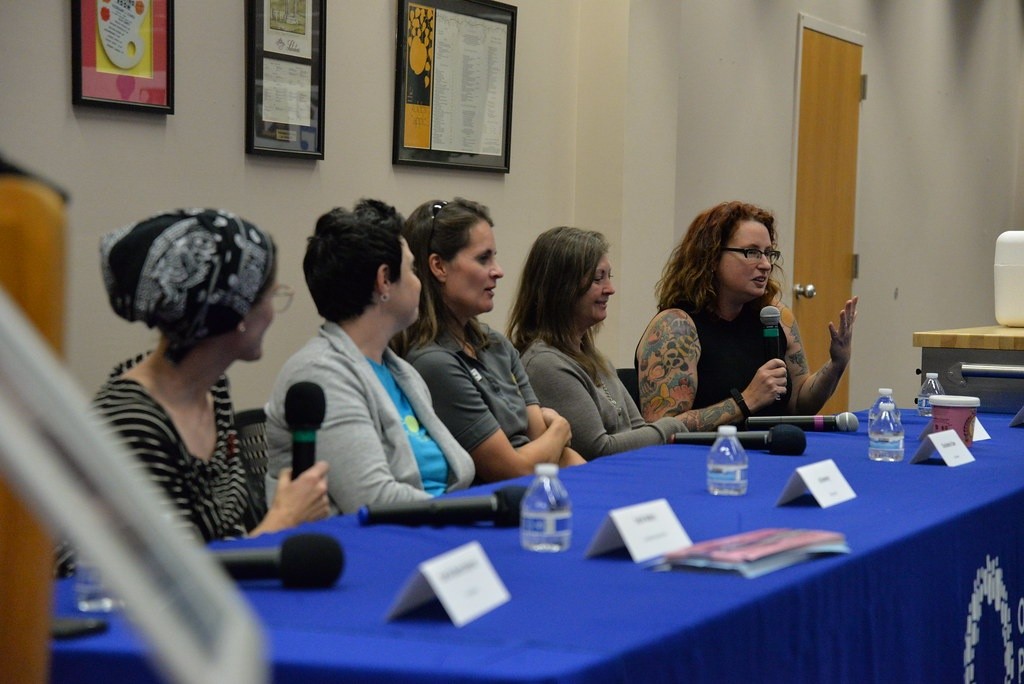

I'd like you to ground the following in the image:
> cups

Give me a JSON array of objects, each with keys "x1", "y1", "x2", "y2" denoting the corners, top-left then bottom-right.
[{"x1": 928, "y1": 393, "x2": 981, "y2": 448}]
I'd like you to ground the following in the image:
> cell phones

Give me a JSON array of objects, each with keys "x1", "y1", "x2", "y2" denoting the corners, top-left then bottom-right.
[{"x1": 50, "y1": 618, "x2": 112, "y2": 639}]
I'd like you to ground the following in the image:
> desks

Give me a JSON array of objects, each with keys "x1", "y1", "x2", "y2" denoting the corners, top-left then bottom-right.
[{"x1": 51, "y1": 408, "x2": 1024, "y2": 684}]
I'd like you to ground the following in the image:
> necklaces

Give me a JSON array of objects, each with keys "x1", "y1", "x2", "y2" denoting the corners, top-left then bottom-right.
[{"x1": 601, "y1": 381, "x2": 616, "y2": 406}]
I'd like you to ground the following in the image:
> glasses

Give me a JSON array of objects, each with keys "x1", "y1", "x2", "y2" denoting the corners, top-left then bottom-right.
[
  {"x1": 258, "y1": 285, "x2": 293, "y2": 312},
  {"x1": 720, "y1": 248, "x2": 780, "y2": 265},
  {"x1": 427, "y1": 201, "x2": 450, "y2": 258}
]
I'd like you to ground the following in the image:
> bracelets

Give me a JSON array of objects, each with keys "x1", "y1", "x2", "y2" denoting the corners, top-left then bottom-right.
[{"x1": 731, "y1": 388, "x2": 751, "y2": 418}]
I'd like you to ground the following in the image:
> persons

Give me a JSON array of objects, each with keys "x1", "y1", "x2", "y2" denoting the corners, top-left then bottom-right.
[
  {"x1": 509, "y1": 226, "x2": 688, "y2": 457},
  {"x1": 51, "y1": 207, "x2": 329, "y2": 578},
  {"x1": 634, "y1": 201, "x2": 860, "y2": 432},
  {"x1": 264, "y1": 198, "x2": 475, "y2": 516},
  {"x1": 389, "y1": 195, "x2": 586, "y2": 476}
]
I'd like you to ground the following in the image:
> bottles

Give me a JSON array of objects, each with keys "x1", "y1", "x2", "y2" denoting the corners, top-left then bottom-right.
[
  {"x1": 519, "y1": 463, "x2": 573, "y2": 552},
  {"x1": 73, "y1": 548, "x2": 121, "y2": 613},
  {"x1": 868, "y1": 403, "x2": 905, "y2": 461},
  {"x1": 918, "y1": 372, "x2": 945, "y2": 417},
  {"x1": 868, "y1": 386, "x2": 899, "y2": 428},
  {"x1": 706, "y1": 425, "x2": 750, "y2": 496}
]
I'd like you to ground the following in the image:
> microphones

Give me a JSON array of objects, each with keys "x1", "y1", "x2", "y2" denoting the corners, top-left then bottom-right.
[
  {"x1": 359, "y1": 485, "x2": 530, "y2": 529},
  {"x1": 744, "y1": 412, "x2": 860, "y2": 431},
  {"x1": 284, "y1": 381, "x2": 326, "y2": 482},
  {"x1": 761, "y1": 307, "x2": 779, "y2": 363},
  {"x1": 205, "y1": 534, "x2": 346, "y2": 592},
  {"x1": 671, "y1": 424, "x2": 806, "y2": 456}
]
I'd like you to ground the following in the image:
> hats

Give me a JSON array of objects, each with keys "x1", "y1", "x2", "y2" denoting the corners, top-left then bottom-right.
[{"x1": 98, "y1": 206, "x2": 277, "y2": 347}]
